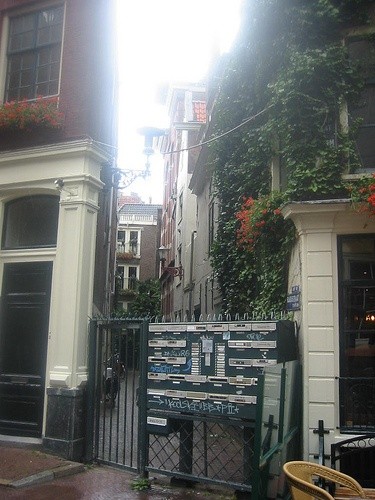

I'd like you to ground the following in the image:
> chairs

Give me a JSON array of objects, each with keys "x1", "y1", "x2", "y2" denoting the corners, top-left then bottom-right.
[{"x1": 282, "y1": 460, "x2": 368, "y2": 500}]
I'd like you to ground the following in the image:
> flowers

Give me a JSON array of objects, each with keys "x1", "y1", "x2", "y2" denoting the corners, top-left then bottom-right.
[
  {"x1": 234, "y1": 196, "x2": 284, "y2": 253},
  {"x1": 347, "y1": 172, "x2": 375, "y2": 219},
  {"x1": 0, "y1": 92, "x2": 65, "y2": 132}
]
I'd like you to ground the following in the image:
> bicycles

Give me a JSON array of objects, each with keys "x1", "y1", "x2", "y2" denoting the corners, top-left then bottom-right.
[{"x1": 103, "y1": 351, "x2": 129, "y2": 407}]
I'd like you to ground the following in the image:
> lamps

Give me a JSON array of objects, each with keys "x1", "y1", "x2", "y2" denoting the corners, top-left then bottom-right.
[{"x1": 156, "y1": 245, "x2": 184, "y2": 278}]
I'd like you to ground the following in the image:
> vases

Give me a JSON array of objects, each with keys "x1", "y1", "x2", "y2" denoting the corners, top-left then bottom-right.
[{"x1": 0, "y1": 122, "x2": 55, "y2": 151}]
